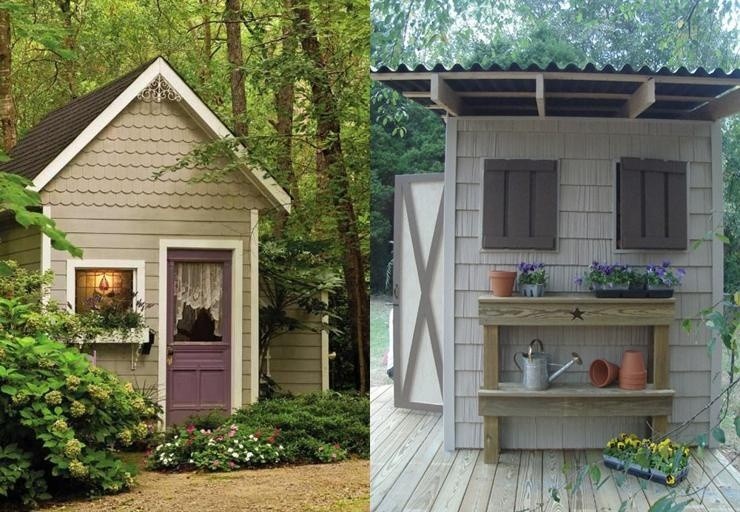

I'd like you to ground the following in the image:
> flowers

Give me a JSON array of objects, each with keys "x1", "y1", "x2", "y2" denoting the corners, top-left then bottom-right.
[
  {"x1": 569, "y1": 257, "x2": 687, "y2": 287},
  {"x1": 607, "y1": 426, "x2": 690, "y2": 485},
  {"x1": 517, "y1": 259, "x2": 548, "y2": 284}
]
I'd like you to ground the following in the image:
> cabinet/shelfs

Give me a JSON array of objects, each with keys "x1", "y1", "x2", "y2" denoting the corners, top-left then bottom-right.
[{"x1": 477, "y1": 295, "x2": 678, "y2": 464}]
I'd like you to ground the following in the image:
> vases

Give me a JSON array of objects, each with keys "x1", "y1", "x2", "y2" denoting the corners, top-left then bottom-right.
[
  {"x1": 490, "y1": 271, "x2": 517, "y2": 296},
  {"x1": 593, "y1": 281, "x2": 674, "y2": 298},
  {"x1": 590, "y1": 347, "x2": 648, "y2": 390},
  {"x1": 523, "y1": 285, "x2": 547, "y2": 295},
  {"x1": 599, "y1": 452, "x2": 688, "y2": 488}
]
